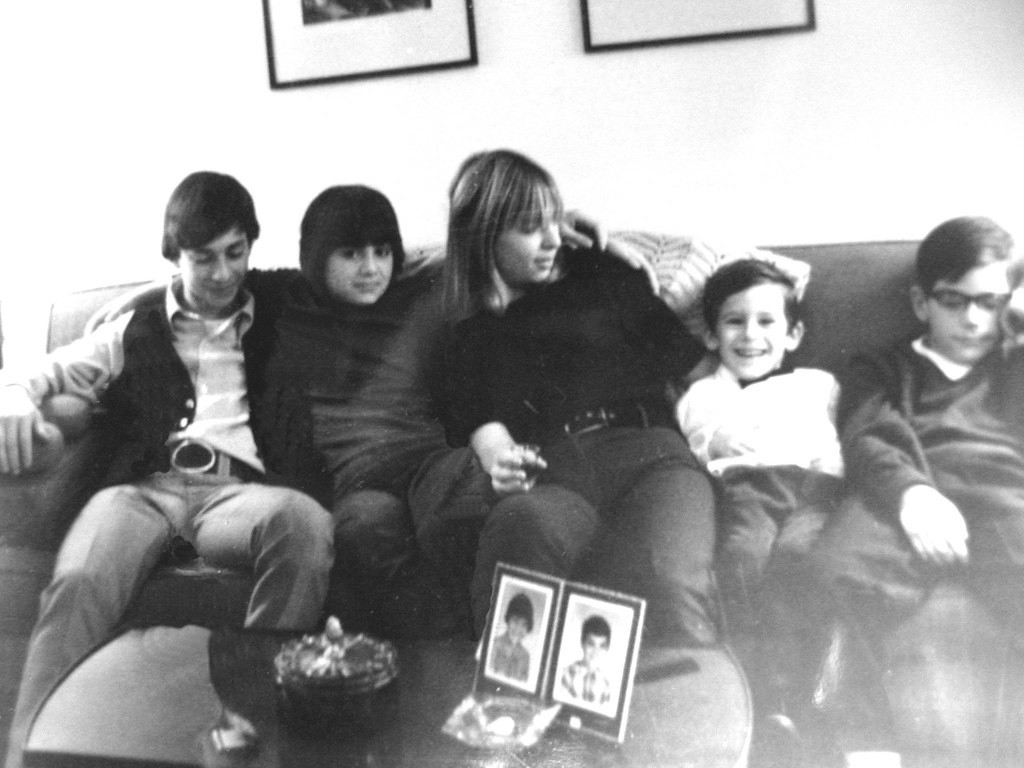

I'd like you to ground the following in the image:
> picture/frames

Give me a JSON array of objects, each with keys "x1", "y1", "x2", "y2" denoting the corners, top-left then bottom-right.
[
  {"x1": 259, "y1": 0, "x2": 478, "y2": 91},
  {"x1": 546, "y1": 582, "x2": 648, "y2": 744},
  {"x1": 474, "y1": 561, "x2": 565, "y2": 717},
  {"x1": 580, "y1": 1, "x2": 815, "y2": 53}
]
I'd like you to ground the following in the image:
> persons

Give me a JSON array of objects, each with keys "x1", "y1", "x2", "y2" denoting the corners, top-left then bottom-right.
[
  {"x1": 760, "y1": 216, "x2": 1023, "y2": 768},
  {"x1": 488, "y1": 591, "x2": 533, "y2": 682},
  {"x1": 4, "y1": 171, "x2": 607, "y2": 763},
  {"x1": 559, "y1": 615, "x2": 614, "y2": 709},
  {"x1": 674, "y1": 258, "x2": 846, "y2": 710},
  {"x1": 84, "y1": 186, "x2": 661, "y2": 643},
  {"x1": 422, "y1": 148, "x2": 720, "y2": 648}
]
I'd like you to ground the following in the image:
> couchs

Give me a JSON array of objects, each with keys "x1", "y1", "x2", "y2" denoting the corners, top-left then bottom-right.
[{"x1": 1, "y1": 240, "x2": 1024, "y2": 733}]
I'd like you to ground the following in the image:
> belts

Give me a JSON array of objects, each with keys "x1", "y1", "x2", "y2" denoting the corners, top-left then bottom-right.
[
  {"x1": 541, "y1": 405, "x2": 677, "y2": 441},
  {"x1": 165, "y1": 439, "x2": 264, "y2": 482}
]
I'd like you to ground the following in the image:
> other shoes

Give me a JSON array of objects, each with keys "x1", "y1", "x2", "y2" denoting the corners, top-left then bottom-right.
[{"x1": 765, "y1": 712, "x2": 808, "y2": 765}]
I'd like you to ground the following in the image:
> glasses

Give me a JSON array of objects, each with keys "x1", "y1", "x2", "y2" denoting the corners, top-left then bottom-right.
[{"x1": 927, "y1": 289, "x2": 1012, "y2": 315}]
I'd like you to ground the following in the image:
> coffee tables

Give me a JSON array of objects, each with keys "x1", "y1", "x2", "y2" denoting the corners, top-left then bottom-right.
[{"x1": 22, "y1": 623, "x2": 756, "y2": 768}]
